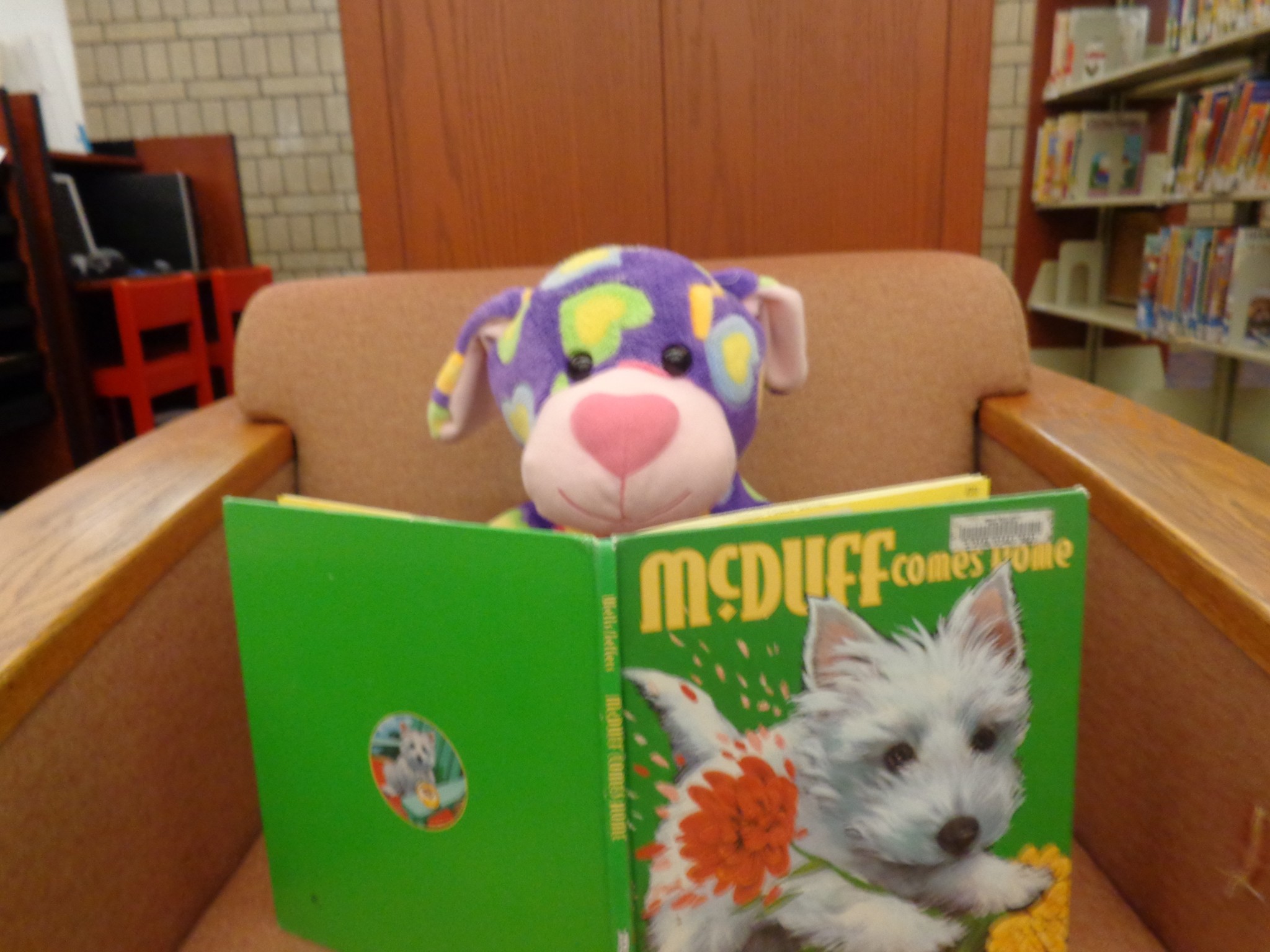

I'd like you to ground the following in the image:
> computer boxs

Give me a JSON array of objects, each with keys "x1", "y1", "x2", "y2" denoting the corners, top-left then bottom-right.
[{"x1": 84, "y1": 172, "x2": 204, "y2": 274}]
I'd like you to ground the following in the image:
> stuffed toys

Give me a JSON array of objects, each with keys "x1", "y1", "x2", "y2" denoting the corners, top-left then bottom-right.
[{"x1": 428, "y1": 243, "x2": 808, "y2": 537}]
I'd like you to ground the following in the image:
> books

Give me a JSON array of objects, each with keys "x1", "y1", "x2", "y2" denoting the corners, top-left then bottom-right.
[
  {"x1": 1160, "y1": 77, "x2": 1270, "y2": 194},
  {"x1": 1033, "y1": 112, "x2": 1148, "y2": 197},
  {"x1": 1051, "y1": 6, "x2": 1150, "y2": 85},
  {"x1": 1165, "y1": 0, "x2": 1270, "y2": 55},
  {"x1": 1136, "y1": 226, "x2": 1270, "y2": 348},
  {"x1": 220, "y1": 472, "x2": 1090, "y2": 952}
]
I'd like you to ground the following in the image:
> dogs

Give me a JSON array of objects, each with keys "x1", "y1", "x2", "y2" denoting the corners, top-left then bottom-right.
[
  {"x1": 625, "y1": 555, "x2": 1054, "y2": 952},
  {"x1": 382, "y1": 719, "x2": 438, "y2": 800}
]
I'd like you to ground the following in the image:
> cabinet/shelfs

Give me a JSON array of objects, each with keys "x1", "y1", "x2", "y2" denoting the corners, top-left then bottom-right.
[
  {"x1": 0, "y1": 91, "x2": 250, "y2": 508},
  {"x1": 1023, "y1": 22, "x2": 1270, "y2": 444}
]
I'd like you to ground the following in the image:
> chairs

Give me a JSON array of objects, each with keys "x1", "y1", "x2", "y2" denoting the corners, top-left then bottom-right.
[{"x1": 0, "y1": 243, "x2": 1270, "y2": 952}]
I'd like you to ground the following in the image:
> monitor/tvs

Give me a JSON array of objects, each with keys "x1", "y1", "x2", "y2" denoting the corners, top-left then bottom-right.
[{"x1": 53, "y1": 173, "x2": 97, "y2": 259}]
